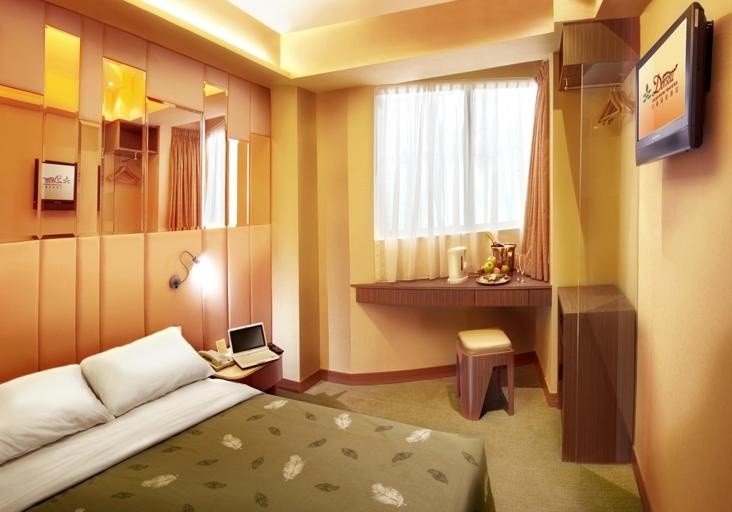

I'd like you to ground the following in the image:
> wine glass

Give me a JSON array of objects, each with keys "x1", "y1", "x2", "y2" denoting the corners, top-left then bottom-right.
[{"x1": 515, "y1": 254, "x2": 528, "y2": 283}]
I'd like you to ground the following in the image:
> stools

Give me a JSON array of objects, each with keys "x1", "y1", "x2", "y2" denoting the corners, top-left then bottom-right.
[{"x1": 455, "y1": 328, "x2": 514, "y2": 421}]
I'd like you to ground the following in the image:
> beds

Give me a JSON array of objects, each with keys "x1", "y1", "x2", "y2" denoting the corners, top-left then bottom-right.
[{"x1": 0, "y1": 377, "x2": 496, "y2": 512}]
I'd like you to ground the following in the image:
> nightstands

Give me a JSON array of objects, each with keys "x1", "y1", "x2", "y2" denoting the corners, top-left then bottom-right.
[{"x1": 212, "y1": 354, "x2": 282, "y2": 395}]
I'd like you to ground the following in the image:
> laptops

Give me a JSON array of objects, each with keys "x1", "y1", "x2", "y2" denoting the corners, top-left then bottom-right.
[{"x1": 227, "y1": 321, "x2": 280, "y2": 370}]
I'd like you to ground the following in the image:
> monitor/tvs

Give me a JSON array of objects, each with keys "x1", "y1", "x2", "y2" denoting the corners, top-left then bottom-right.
[
  {"x1": 634, "y1": 1, "x2": 713, "y2": 168},
  {"x1": 33, "y1": 159, "x2": 78, "y2": 210}
]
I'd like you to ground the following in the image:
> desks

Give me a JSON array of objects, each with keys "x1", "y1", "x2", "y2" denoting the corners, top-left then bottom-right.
[{"x1": 350, "y1": 269, "x2": 552, "y2": 307}]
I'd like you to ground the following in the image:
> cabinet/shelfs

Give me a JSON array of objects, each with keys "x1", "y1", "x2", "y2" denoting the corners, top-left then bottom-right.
[
  {"x1": 558, "y1": 17, "x2": 641, "y2": 91},
  {"x1": 557, "y1": 284, "x2": 636, "y2": 465}
]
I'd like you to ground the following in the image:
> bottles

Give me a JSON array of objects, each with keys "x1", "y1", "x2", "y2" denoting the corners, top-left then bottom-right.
[{"x1": 486, "y1": 232, "x2": 505, "y2": 247}]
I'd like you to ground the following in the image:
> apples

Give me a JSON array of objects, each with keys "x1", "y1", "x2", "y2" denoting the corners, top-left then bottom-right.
[
  {"x1": 487, "y1": 255, "x2": 496, "y2": 267},
  {"x1": 483, "y1": 262, "x2": 493, "y2": 273},
  {"x1": 501, "y1": 264, "x2": 510, "y2": 274}
]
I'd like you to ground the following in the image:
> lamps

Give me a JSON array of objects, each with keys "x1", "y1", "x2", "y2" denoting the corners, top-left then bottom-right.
[{"x1": 169, "y1": 252, "x2": 199, "y2": 290}]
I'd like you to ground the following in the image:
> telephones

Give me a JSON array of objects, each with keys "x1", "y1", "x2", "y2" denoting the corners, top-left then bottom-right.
[{"x1": 198, "y1": 349, "x2": 234, "y2": 372}]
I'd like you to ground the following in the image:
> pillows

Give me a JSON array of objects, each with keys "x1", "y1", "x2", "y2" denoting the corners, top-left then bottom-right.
[
  {"x1": 79, "y1": 325, "x2": 216, "y2": 417},
  {"x1": 0, "y1": 364, "x2": 116, "y2": 465}
]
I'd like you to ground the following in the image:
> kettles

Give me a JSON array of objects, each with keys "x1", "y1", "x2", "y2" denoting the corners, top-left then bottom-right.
[{"x1": 447, "y1": 246, "x2": 469, "y2": 284}]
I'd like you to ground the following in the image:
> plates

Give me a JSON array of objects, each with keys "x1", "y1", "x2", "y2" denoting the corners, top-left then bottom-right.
[{"x1": 475, "y1": 273, "x2": 512, "y2": 285}]
